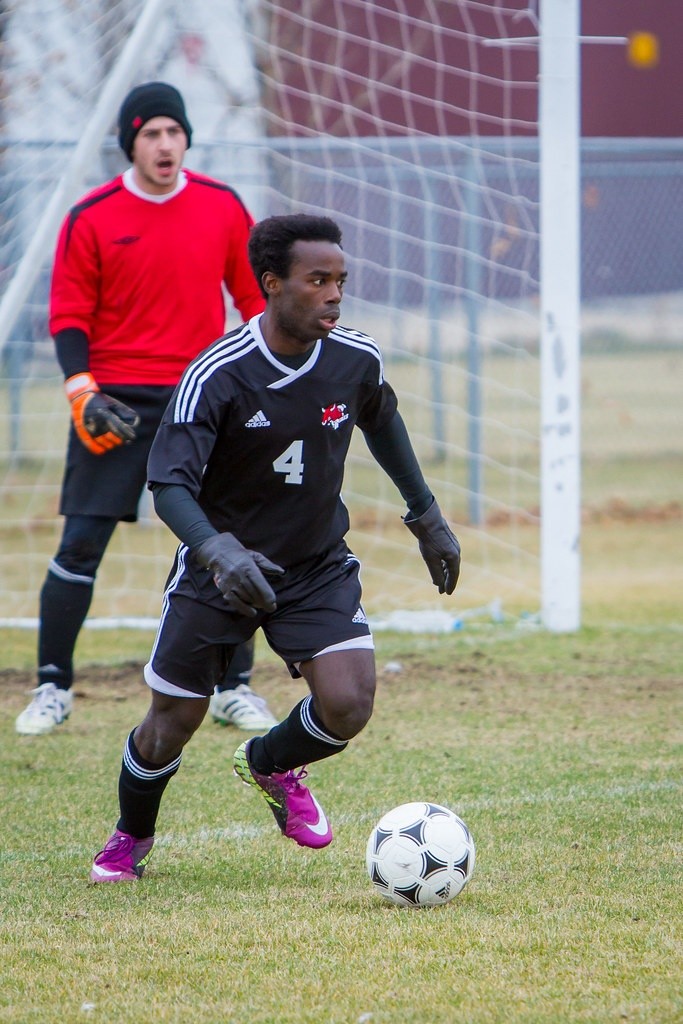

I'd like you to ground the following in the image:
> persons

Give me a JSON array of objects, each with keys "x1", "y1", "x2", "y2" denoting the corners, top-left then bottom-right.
[
  {"x1": 92, "y1": 214, "x2": 460, "y2": 881},
  {"x1": 17, "y1": 82, "x2": 279, "y2": 732}
]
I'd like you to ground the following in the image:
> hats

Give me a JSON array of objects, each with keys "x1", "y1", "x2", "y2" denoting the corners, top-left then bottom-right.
[{"x1": 116, "y1": 82, "x2": 192, "y2": 164}]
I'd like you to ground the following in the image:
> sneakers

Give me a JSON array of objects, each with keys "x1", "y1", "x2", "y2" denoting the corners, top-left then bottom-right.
[
  {"x1": 15, "y1": 682, "x2": 73, "y2": 736},
  {"x1": 210, "y1": 684, "x2": 278, "y2": 732},
  {"x1": 87, "y1": 828, "x2": 154, "y2": 886},
  {"x1": 234, "y1": 736, "x2": 333, "y2": 850}
]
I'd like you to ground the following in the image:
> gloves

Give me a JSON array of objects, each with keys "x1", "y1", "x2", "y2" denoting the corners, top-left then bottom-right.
[
  {"x1": 64, "y1": 372, "x2": 139, "y2": 454},
  {"x1": 197, "y1": 533, "x2": 284, "y2": 617},
  {"x1": 402, "y1": 496, "x2": 460, "y2": 595}
]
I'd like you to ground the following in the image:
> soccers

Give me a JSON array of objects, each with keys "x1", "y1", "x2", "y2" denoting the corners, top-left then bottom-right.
[{"x1": 365, "y1": 800, "x2": 476, "y2": 909}]
